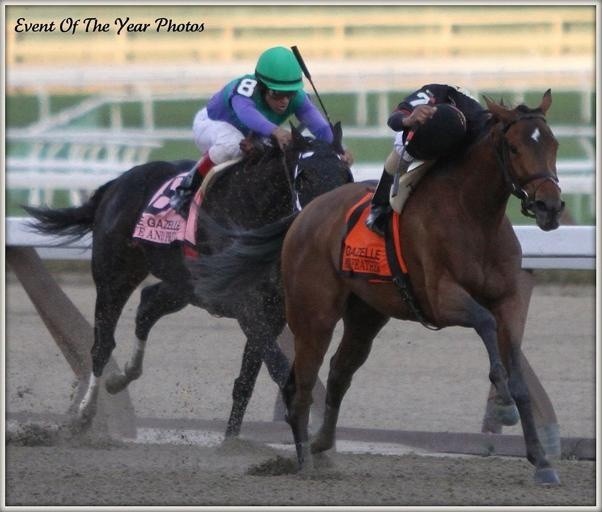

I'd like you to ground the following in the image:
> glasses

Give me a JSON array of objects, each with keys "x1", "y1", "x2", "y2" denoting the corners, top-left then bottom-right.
[{"x1": 257, "y1": 80, "x2": 296, "y2": 99}]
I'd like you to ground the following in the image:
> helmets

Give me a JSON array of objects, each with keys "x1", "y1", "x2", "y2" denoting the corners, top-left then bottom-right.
[
  {"x1": 255, "y1": 47, "x2": 304, "y2": 91},
  {"x1": 413, "y1": 103, "x2": 467, "y2": 164}
]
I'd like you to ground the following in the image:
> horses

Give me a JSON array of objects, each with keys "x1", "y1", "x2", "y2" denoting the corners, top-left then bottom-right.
[
  {"x1": 185, "y1": 87, "x2": 566, "y2": 487},
  {"x1": 16, "y1": 119, "x2": 354, "y2": 439}
]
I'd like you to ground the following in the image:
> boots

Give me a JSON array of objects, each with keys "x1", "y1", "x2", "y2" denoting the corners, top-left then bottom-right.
[
  {"x1": 365, "y1": 164, "x2": 398, "y2": 236},
  {"x1": 170, "y1": 165, "x2": 203, "y2": 219}
]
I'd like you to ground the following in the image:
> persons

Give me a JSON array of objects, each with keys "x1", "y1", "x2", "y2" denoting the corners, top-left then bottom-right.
[
  {"x1": 170, "y1": 47, "x2": 354, "y2": 221},
  {"x1": 364, "y1": 84, "x2": 490, "y2": 238}
]
